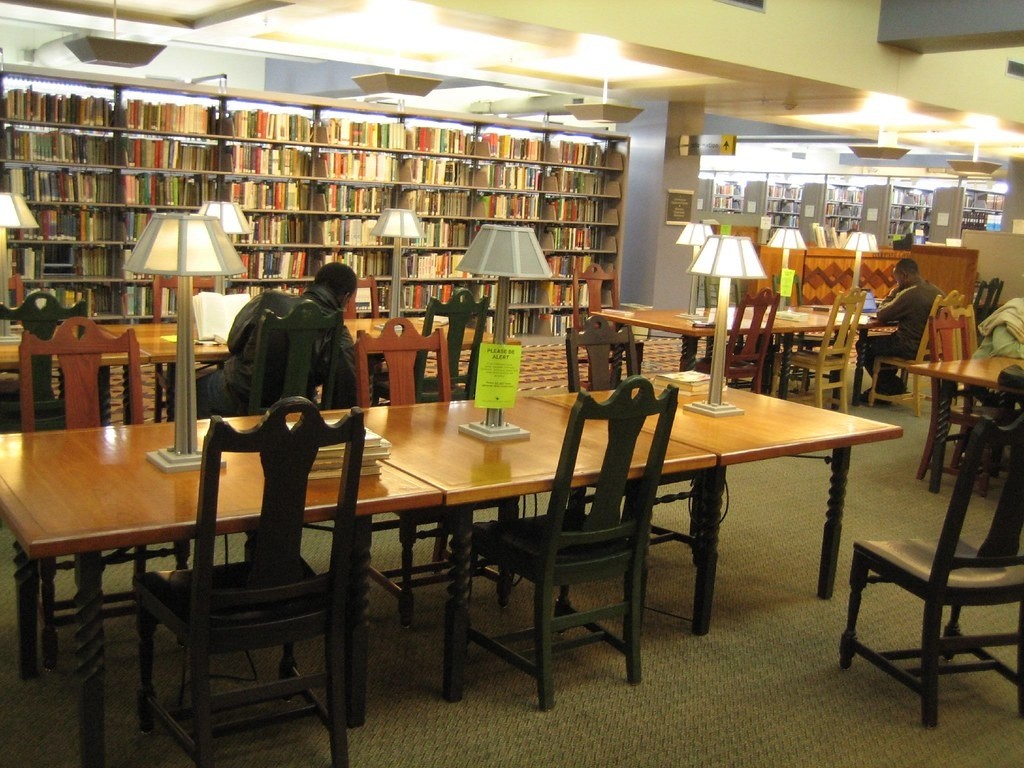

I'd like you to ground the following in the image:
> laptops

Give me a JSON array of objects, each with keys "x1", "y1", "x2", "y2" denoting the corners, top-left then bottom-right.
[{"x1": 855, "y1": 288, "x2": 879, "y2": 318}]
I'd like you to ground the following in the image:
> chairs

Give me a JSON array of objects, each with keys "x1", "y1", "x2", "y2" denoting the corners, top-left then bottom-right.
[
  {"x1": 459, "y1": 374, "x2": 680, "y2": 712},
  {"x1": 703, "y1": 275, "x2": 775, "y2": 396},
  {"x1": 772, "y1": 286, "x2": 867, "y2": 414},
  {"x1": 127, "y1": 393, "x2": 367, "y2": 768},
  {"x1": 915, "y1": 307, "x2": 1005, "y2": 496},
  {"x1": 564, "y1": 314, "x2": 702, "y2": 566},
  {"x1": 573, "y1": 263, "x2": 645, "y2": 390},
  {"x1": 869, "y1": 290, "x2": 966, "y2": 417},
  {"x1": 973, "y1": 276, "x2": 1005, "y2": 343},
  {"x1": 19, "y1": 316, "x2": 191, "y2": 677},
  {"x1": 354, "y1": 316, "x2": 521, "y2": 632},
  {"x1": 772, "y1": 273, "x2": 836, "y2": 393},
  {"x1": 944, "y1": 304, "x2": 1003, "y2": 469},
  {"x1": 693, "y1": 286, "x2": 781, "y2": 393},
  {"x1": 837, "y1": 413, "x2": 1024, "y2": 731}
]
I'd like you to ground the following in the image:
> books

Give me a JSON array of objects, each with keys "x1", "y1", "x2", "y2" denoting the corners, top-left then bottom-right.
[
  {"x1": 713, "y1": 185, "x2": 741, "y2": 209},
  {"x1": 768, "y1": 186, "x2": 802, "y2": 227},
  {"x1": 961, "y1": 194, "x2": 1005, "y2": 231},
  {"x1": 825, "y1": 188, "x2": 864, "y2": 231},
  {"x1": 654, "y1": 370, "x2": 726, "y2": 392},
  {"x1": 286, "y1": 421, "x2": 392, "y2": 480},
  {"x1": 0, "y1": 89, "x2": 601, "y2": 337},
  {"x1": 889, "y1": 189, "x2": 928, "y2": 234}
]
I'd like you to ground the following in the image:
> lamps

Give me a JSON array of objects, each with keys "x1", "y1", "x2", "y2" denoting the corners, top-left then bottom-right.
[
  {"x1": 946, "y1": 141, "x2": 1002, "y2": 174},
  {"x1": 766, "y1": 227, "x2": 808, "y2": 311},
  {"x1": 563, "y1": 78, "x2": 645, "y2": 123},
  {"x1": 847, "y1": 127, "x2": 911, "y2": 161},
  {"x1": 0, "y1": 192, "x2": 41, "y2": 347},
  {"x1": 675, "y1": 222, "x2": 714, "y2": 320},
  {"x1": 196, "y1": 200, "x2": 254, "y2": 295},
  {"x1": 62, "y1": 0, "x2": 167, "y2": 69},
  {"x1": 370, "y1": 207, "x2": 428, "y2": 333},
  {"x1": 844, "y1": 231, "x2": 879, "y2": 286},
  {"x1": 453, "y1": 224, "x2": 555, "y2": 446},
  {"x1": 684, "y1": 234, "x2": 768, "y2": 418},
  {"x1": 351, "y1": 50, "x2": 444, "y2": 98},
  {"x1": 119, "y1": 211, "x2": 248, "y2": 476}
]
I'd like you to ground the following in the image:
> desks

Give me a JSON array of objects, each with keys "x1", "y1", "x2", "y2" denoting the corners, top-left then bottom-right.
[
  {"x1": 804, "y1": 304, "x2": 909, "y2": 405},
  {"x1": 535, "y1": 386, "x2": 904, "y2": 638},
  {"x1": 0, "y1": 326, "x2": 152, "y2": 426},
  {"x1": 294, "y1": 395, "x2": 719, "y2": 704},
  {"x1": 699, "y1": 306, "x2": 875, "y2": 405},
  {"x1": 589, "y1": 307, "x2": 793, "y2": 407},
  {"x1": 907, "y1": 356, "x2": 1024, "y2": 492},
  {"x1": 0, "y1": 413, "x2": 442, "y2": 768},
  {"x1": 110, "y1": 323, "x2": 355, "y2": 418},
  {"x1": 347, "y1": 316, "x2": 521, "y2": 405}
]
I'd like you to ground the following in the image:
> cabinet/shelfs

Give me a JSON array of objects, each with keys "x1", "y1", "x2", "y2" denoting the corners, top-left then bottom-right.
[
  {"x1": 929, "y1": 186, "x2": 1005, "y2": 246},
  {"x1": 800, "y1": 182, "x2": 864, "y2": 242},
  {"x1": 0, "y1": 62, "x2": 632, "y2": 345},
  {"x1": 698, "y1": 177, "x2": 744, "y2": 213},
  {"x1": 741, "y1": 180, "x2": 802, "y2": 229},
  {"x1": 860, "y1": 184, "x2": 933, "y2": 246}
]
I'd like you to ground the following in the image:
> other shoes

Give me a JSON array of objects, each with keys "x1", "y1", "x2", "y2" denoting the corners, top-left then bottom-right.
[{"x1": 864, "y1": 387, "x2": 907, "y2": 405}]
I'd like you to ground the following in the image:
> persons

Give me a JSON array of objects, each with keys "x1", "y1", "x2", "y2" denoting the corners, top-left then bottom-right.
[
  {"x1": 196, "y1": 262, "x2": 358, "y2": 418},
  {"x1": 856, "y1": 258, "x2": 945, "y2": 397},
  {"x1": 971, "y1": 297, "x2": 1024, "y2": 407}
]
